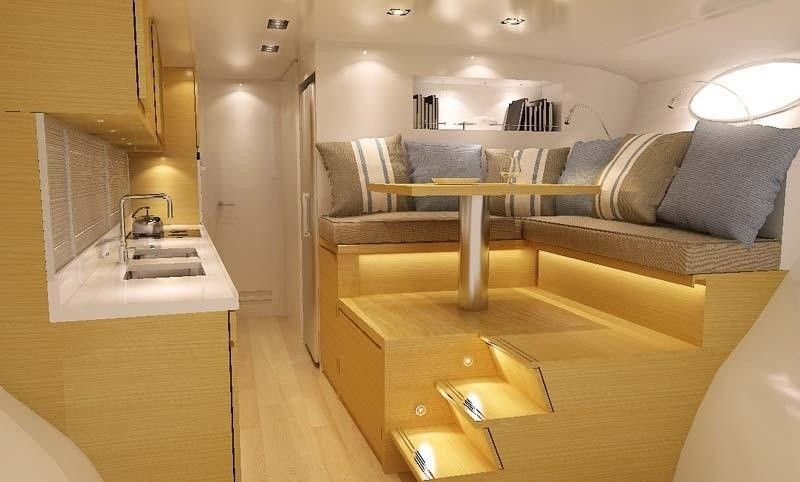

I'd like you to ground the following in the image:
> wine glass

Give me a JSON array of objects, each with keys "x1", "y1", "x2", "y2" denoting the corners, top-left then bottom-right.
[{"x1": 500, "y1": 156, "x2": 522, "y2": 183}]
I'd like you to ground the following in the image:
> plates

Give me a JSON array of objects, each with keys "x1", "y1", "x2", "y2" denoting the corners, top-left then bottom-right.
[{"x1": 431, "y1": 177, "x2": 480, "y2": 184}]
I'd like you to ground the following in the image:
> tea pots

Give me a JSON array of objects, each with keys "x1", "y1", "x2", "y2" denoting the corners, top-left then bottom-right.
[{"x1": 132, "y1": 206, "x2": 164, "y2": 235}]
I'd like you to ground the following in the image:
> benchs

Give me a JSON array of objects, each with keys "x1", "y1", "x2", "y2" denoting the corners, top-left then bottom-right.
[{"x1": 314, "y1": 210, "x2": 791, "y2": 350}]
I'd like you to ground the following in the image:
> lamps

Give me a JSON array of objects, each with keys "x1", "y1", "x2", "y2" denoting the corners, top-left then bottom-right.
[
  {"x1": 667, "y1": 80, "x2": 753, "y2": 123},
  {"x1": 564, "y1": 104, "x2": 611, "y2": 140}
]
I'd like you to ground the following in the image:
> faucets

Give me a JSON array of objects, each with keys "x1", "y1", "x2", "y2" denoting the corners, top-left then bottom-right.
[{"x1": 120, "y1": 193, "x2": 174, "y2": 237}]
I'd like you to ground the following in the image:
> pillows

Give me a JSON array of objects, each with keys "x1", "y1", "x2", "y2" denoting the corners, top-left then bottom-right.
[
  {"x1": 593, "y1": 130, "x2": 692, "y2": 224},
  {"x1": 555, "y1": 136, "x2": 619, "y2": 216},
  {"x1": 657, "y1": 119, "x2": 799, "y2": 244},
  {"x1": 486, "y1": 147, "x2": 570, "y2": 216},
  {"x1": 315, "y1": 134, "x2": 411, "y2": 218}
]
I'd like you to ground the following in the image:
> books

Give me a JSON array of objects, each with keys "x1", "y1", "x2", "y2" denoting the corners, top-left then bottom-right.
[
  {"x1": 412, "y1": 93, "x2": 440, "y2": 130},
  {"x1": 505, "y1": 97, "x2": 555, "y2": 132}
]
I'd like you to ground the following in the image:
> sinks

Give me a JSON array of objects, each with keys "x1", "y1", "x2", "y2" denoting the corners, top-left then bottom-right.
[
  {"x1": 131, "y1": 248, "x2": 200, "y2": 260},
  {"x1": 123, "y1": 262, "x2": 206, "y2": 280}
]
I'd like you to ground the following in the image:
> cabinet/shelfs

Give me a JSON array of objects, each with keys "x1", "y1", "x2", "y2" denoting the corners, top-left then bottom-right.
[{"x1": 0, "y1": 0, "x2": 241, "y2": 481}]
